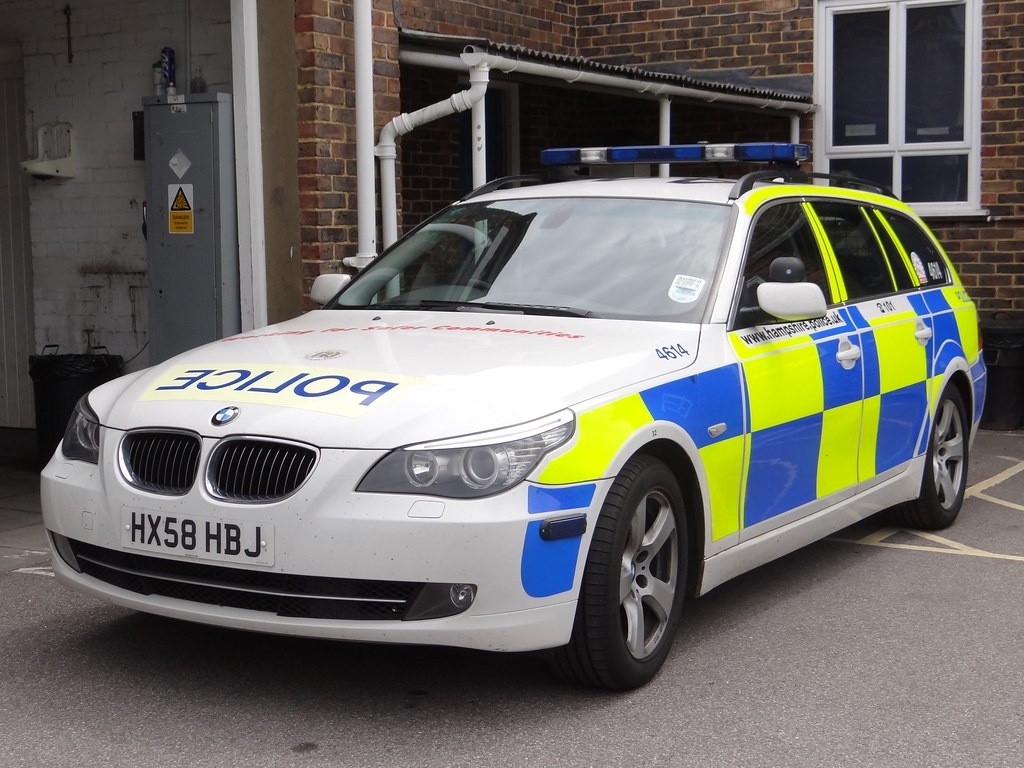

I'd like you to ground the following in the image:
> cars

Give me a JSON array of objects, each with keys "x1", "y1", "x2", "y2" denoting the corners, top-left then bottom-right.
[{"x1": 39, "y1": 142, "x2": 988, "y2": 693}]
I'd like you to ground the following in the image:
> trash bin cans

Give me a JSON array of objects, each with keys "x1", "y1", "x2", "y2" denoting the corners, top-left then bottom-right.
[
  {"x1": 30, "y1": 344, "x2": 123, "y2": 479},
  {"x1": 979, "y1": 308, "x2": 1024, "y2": 431}
]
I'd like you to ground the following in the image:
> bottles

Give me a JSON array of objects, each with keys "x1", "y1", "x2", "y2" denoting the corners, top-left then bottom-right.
[
  {"x1": 190, "y1": 66, "x2": 206, "y2": 93},
  {"x1": 152, "y1": 46, "x2": 175, "y2": 97}
]
